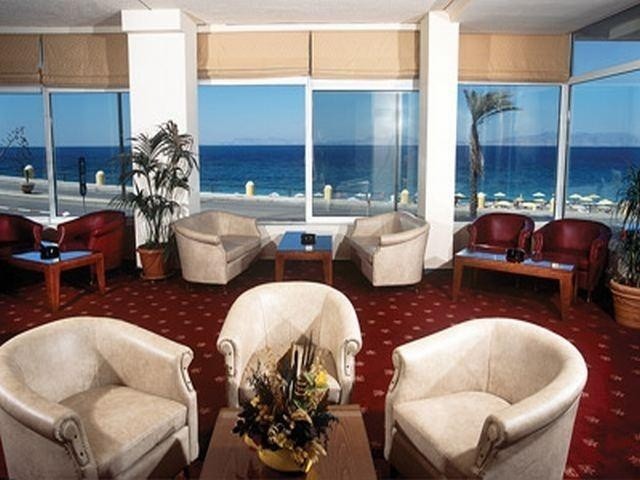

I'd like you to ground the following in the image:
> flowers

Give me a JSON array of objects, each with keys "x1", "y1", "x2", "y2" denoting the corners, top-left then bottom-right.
[{"x1": 232, "y1": 329, "x2": 339, "y2": 465}]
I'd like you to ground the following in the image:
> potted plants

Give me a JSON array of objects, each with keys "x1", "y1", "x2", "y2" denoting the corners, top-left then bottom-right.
[
  {"x1": 607, "y1": 160, "x2": 640, "y2": 329},
  {"x1": 104, "y1": 119, "x2": 201, "y2": 282}
]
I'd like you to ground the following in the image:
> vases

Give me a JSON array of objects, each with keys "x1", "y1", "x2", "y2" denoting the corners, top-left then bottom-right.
[{"x1": 242, "y1": 433, "x2": 313, "y2": 474}]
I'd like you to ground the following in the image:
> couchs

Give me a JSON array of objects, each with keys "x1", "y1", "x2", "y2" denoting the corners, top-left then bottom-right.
[
  {"x1": 0, "y1": 212, "x2": 43, "y2": 283},
  {"x1": 348, "y1": 209, "x2": 431, "y2": 294},
  {"x1": 532, "y1": 218, "x2": 612, "y2": 303},
  {"x1": 0, "y1": 315, "x2": 200, "y2": 480},
  {"x1": 171, "y1": 209, "x2": 263, "y2": 295},
  {"x1": 216, "y1": 279, "x2": 363, "y2": 408},
  {"x1": 56, "y1": 209, "x2": 125, "y2": 286},
  {"x1": 467, "y1": 211, "x2": 534, "y2": 289},
  {"x1": 382, "y1": 316, "x2": 588, "y2": 480}
]
